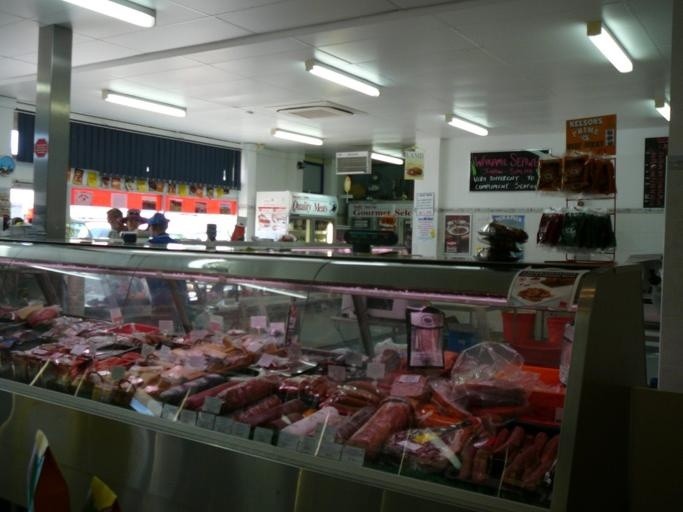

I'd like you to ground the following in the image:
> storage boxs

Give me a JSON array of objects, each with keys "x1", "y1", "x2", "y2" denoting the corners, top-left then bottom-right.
[{"x1": 444, "y1": 321, "x2": 477, "y2": 353}]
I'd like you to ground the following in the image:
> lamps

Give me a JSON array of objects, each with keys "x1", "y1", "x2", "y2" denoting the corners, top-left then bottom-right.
[
  {"x1": 303, "y1": 60, "x2": 382, "y2": 99},
  {"x1": 585, "y1": 19, "x2": 638, "y2": 73},
  {"x1": 442, "y1": 113, "x2": 490, "y2": 139},
  {"x1": 54, "y1": 0, "x2": 156, "y2": 28},
  {"x1": 368, "y1": 150, "x2": 404, "y2": 166},
  {"x1": 96, "y1": 88, "x2": 185, "y2": 120},
  {"x1": 268, "y1": 128, "x2": 324, "y2": 147},
  {"x1": 653, "y1": 96, "x2": 669, "y2": 126}
]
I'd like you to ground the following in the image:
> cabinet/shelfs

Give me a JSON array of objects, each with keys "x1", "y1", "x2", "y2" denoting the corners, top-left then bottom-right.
[
  {"x1": 253, "y1": 192, "x2": 338, "y2": 243},
  {"x1": 0, "y1": 229, "x2": 651, "y2": 511}
]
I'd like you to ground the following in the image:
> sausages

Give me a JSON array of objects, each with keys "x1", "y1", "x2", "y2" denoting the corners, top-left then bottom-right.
[{"x1": 459, "y1": 425, "x2": 558, "y2": 495}]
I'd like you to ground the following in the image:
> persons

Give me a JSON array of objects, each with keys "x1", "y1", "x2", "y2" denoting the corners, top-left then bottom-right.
[
  {"x1": 106, "y1": 208, "x2": 124, "y2": 239},
  {"x1": 120, "y1": 209, "x2": 150, "y2": 235},
  {"x1": 146, "y1": 213, "x2": 187, "y2": 306}
]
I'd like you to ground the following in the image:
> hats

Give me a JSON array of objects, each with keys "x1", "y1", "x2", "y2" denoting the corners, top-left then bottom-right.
[
  {"x1": 148, "y1": 214, "x2": 169, "y2": 225},
  {"x1": 118, "y1": 209, "x2": 148, "y2": 223}
]
